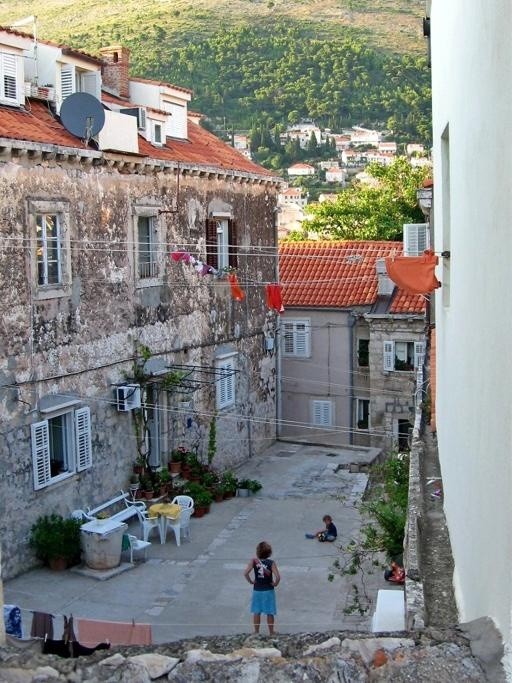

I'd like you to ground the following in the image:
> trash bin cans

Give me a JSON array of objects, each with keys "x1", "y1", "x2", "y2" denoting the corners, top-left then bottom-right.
[{"x1": 80, "y1": 520, "x2": 129, "y2": 570}]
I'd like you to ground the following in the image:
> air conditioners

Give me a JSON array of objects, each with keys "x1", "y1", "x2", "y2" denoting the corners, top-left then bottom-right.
[{"x1": 117, "y1": 382, "x2": 141, "y2": 412}]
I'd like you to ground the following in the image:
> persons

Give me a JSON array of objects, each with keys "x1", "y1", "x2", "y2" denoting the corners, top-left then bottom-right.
[
  {"x1": 244, "y1": 541, "x2": 281, "y2": 634},
  {"x1": 305, "y1": 514, "x2": 337, "y2": 542}
]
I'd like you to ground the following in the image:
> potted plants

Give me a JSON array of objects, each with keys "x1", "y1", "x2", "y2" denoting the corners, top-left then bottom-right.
[
  {"x1": 24, "y1": 511, "x2": 87, "y2": 570},
  {"x1": 129, "y1": 447, "x2": 264, "y2": 518}
]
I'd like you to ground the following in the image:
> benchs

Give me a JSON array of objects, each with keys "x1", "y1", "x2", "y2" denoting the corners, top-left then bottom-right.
[{"x1": 81, "y1": 488, "x2": 152, "y2": 563}]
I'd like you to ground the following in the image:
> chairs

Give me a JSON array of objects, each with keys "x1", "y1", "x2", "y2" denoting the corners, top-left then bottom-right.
[{"x1": 134, "y1": 495, "x2": 194, "y2": 548}]
